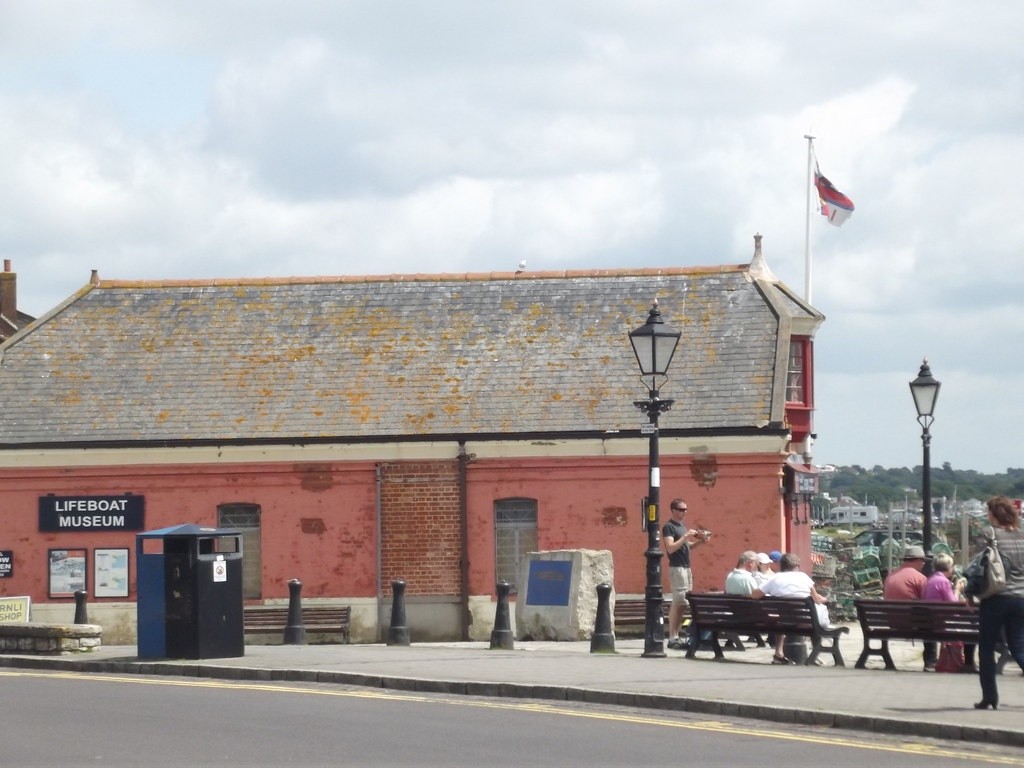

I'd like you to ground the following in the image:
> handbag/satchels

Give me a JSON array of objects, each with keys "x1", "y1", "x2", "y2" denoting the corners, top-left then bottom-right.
[
  {"x1": 935, "y1": 641, "x2": 963, "y2": 672},
  {"x1": 964, "y1": 527, "x2": 1006, "y2": 600},
  {"x1": 815, "y1": 602, "x2": 830, "y2": 627}
]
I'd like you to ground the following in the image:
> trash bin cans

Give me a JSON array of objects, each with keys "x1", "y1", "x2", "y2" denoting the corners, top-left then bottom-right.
[
  {"x1": 165, "y1": 523, "x2": 246, "y2": 661},
  {"x1": 135, "y1": 525, "x2": 186, "y2": 660}
]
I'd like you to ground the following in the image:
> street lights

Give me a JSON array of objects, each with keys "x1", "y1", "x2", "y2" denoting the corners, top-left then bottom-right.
[
  {"x1": 628, "y1": 297, "x2": 683, "y2": 658},
  {"x1": 909, "y1": 358, "x2": 942, "y2": 672}
]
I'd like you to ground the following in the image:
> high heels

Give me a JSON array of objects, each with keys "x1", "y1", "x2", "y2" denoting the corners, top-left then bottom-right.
[{"x1": 974, "y1": 699, "x2": 998, "y2": 710}]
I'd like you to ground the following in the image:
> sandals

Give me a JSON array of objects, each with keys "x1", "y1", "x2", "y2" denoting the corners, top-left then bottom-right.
[{"x1": 772, "y1": 654, "x2": 795, "y2": 664}]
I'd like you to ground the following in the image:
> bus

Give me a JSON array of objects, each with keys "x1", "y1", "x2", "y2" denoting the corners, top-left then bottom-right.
[{"x1": 830, "y1": 506, "x2": 878, "y2": 527}]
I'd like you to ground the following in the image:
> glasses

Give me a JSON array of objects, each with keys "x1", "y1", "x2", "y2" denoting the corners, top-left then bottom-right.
[{"x1": 675, "y1": 508, "x2": 687, "y2": 512}]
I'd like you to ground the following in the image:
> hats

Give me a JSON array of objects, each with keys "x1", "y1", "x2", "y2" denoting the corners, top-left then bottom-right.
[
  {"x1": 898, "y1": 546, "x2": 933, "y2": 560},
  {"x1": 769, "y1": 551, "x2": 782, "y2": 561},
  {"x1": 757, "y1": 553, "x2": 773, "y2": 564}
]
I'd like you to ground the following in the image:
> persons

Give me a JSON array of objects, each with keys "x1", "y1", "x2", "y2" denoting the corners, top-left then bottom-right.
[
  {"x1": 662, "y1": 498, "x2": 712, "y2": 651},
  {"x1": 883, "y1": 546, "x2": 939, "y2": 672},
  {"x1": 975, "y1": 495, "x2": 1024, "y2": 710},
  {"x1": 724, "y1": 550, "x2": 832, "y2": 667},
  {"x1": 921, "y1": 552, "x2": 980, "y2": 673}
]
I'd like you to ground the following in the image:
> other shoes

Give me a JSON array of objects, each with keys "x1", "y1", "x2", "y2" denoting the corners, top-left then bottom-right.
[
  {"x1": 923, "y1": 661, "x2": 937, "y2": 672},
  {"x1": 668, "y1": 638, "x2": 689, "y2": 649}
]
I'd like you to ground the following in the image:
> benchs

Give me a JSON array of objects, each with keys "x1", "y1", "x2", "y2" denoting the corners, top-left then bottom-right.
[
  {"x1": 684, "y1": 593, "x2": 851, "y2": 666},
  {"x1": 614, "y1": 598, "x2": 693, "y2": 626},
  {"x1": 854, "y1": 599, "x2": 1017, "y2": 675},
  {"x1": 243, "y1": 606, "x2": 352, "y2": 643}
]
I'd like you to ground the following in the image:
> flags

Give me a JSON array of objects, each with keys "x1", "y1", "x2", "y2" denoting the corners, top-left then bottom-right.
[{"x1": 811, "y1": 142, "x2": 856, "y2": 229}]
